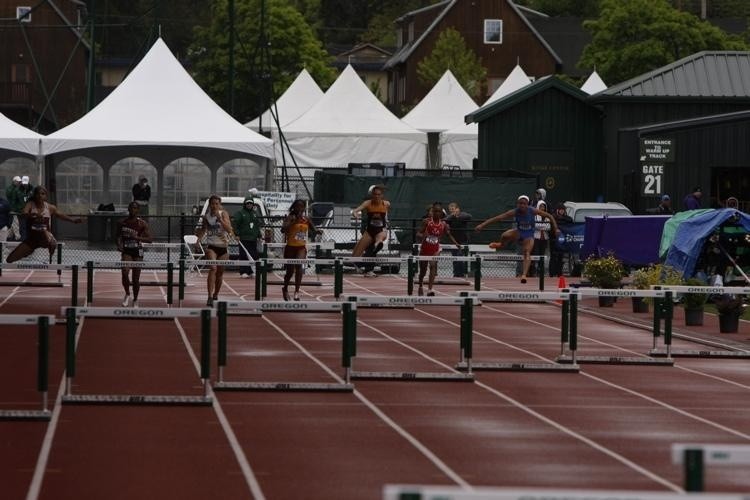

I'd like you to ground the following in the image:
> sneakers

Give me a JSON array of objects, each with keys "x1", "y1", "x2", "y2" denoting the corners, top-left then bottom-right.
[
  {"x1": 365, "y1": 271, "x2": 377, "y2": 277},
  {"x1": 123, "y1": 295, "x2": 130, "y2": 307}
]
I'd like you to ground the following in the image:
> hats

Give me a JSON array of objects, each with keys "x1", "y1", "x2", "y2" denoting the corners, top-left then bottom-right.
[{"x1": 13, "y1": 176, "x2": 29, "y2": 184}]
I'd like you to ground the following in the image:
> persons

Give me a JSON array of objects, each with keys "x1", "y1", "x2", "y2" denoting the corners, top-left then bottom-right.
[
  {"x1": 416, "y1": 186, "x2": 573, "y2": 296},
  {"x1": 683, "y1": 186, "x2": 703, "y2": 210},
  {"x1": 0, "y1": 173, "x2": 82, "y2": 269},
  {"x1": 351, "y1": 187, "x2": 392, "y2": 259},
  {"x1": 231, "y1": 195, "x2": 266, "y2": 278},
  {"x1": 360, "y1": 184, "x2": 381, "y2": 278},
  {"x1": 197, "y1": 195, "x2": 233, "y2": 302},
  {"x1": 117, "y1": 201, "x2": 153, "y2": 307},
  {"x1": 281, "y1": 199, "x2": 323, "y2": 302},
  {"x1": 655, "y1": 194, "x2": 673, "y2": 215}
]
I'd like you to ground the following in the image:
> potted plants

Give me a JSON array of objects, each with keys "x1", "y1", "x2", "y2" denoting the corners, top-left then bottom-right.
[
  {"x1": 627, "y1": 270, "x2": 655, "y2": 313},
  {"x1": 713, "y1": 292, "x2": 745, "y2": 333},
  {"x1": 645, "y1": 263, "x2": 686, "y2": 320},
  {"x1": 583, "y1": 251, "x2": 625, "y2": 308},
  {"x1": 679, "y1": 280, "x2": 710, "y2": 327}
]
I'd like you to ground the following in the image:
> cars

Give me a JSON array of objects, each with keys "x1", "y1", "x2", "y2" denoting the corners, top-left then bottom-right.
[
  {"x1": 564, "y1": 200, "x2": 633, "y2": 275},
  {"x1": 305, "y1": 201, "x2": 402, "y2": 274},
  {"x1": 186, "y1": 196, "x2": 274, "y2": 263}
]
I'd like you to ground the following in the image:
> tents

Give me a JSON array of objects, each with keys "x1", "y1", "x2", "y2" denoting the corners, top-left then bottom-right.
[
  {"x1": 658, "y1": 208, "x2": 750, "y2": 304},
  {"x1": 2, "y1": 36, "x2": 612, "y2": 250}
]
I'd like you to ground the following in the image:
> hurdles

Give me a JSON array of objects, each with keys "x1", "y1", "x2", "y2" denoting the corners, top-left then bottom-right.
[
  {"x1": 60, "y1": 305, "x2": 217, "y2": 407},
  {"x1": 472, "y1": 255, "x2": 547, "y2": 305},
  {"x1": 259, "y1": 257, "x2": 343, "y2": 304},
  {"x1": 86, "y1": 260, "x2": 174, "y2": 307},
  {"x1": 401, "y1": 256, "x2": 482, "y2": 307},
  {"x1": 178, "y1": 259, "x2": 265, "y2": 316},
  {"x1": 555, "y1": 289, "x2": 676, "y2": 367},
  {"x1": 1, "y1": 313, "x2": 57, "y2": 418},
  {"x1": 212, "y1": 300, "x2": 356, "y2": 391},
  {"x1": 455, "y1": 288, "x2": 581, "y2": 373},
  {"x1": 649, "y1": 284, "x2": 750, "y2": 358},
  {"x1": 412, "y1": 243, "x2": 496, "y2": 284},
  {"x1": 337, "y1": 292, "x2": 476, "y2": 382},
  {"x1": 3, "y1": 262, "x2": 81, "y2": 324},
  {"x1": 336, "y1": 256, "x2": 415, "y2": 310}
]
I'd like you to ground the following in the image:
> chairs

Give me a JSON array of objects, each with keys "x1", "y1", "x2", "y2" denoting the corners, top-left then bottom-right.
[{"x1": 180, "y1": 234, "x2": 206, "y2": 279}]
